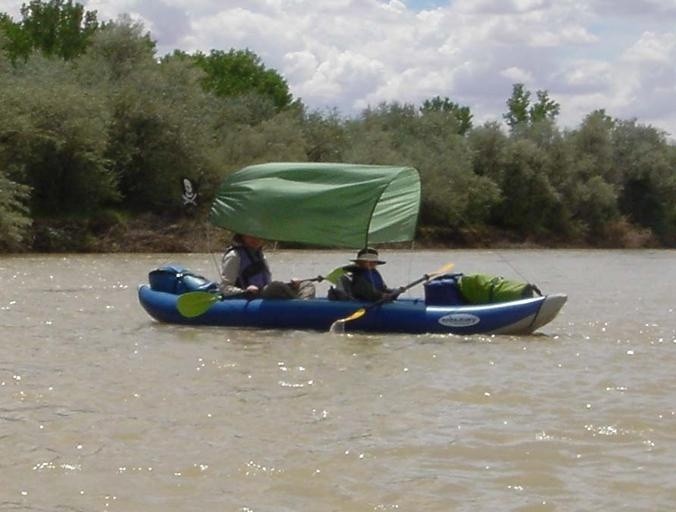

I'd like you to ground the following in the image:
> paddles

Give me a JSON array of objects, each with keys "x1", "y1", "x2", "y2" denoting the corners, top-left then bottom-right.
[
  {"x1": 176, "y1": 266, "x2": 348, "y2": 318},
  {"x1": 338, "y1": 263, "x2": 453, "y2": 323}
]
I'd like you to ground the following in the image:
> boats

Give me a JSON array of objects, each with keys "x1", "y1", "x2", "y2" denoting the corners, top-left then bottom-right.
[{"x1": 136, "y1": 160, "x2": 570, "y2": 336}]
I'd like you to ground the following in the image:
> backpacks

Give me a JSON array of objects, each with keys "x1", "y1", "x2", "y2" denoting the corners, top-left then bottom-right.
[{"x1": 455, "y1": 271, "x2": 538, "y2": 305}]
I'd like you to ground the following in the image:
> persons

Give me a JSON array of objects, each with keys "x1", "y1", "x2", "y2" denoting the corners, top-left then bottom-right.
[
  {"x1": 220, "y1": 233, "x2": 316, "y2": 300},
  {"x1": 348, "y1": 246, "x2": 406, "y2": 303}
]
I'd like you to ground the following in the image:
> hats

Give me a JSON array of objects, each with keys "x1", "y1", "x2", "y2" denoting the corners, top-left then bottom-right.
[{"x1": 349, "y1": 245, "x2": 387, "y2": 265}]
